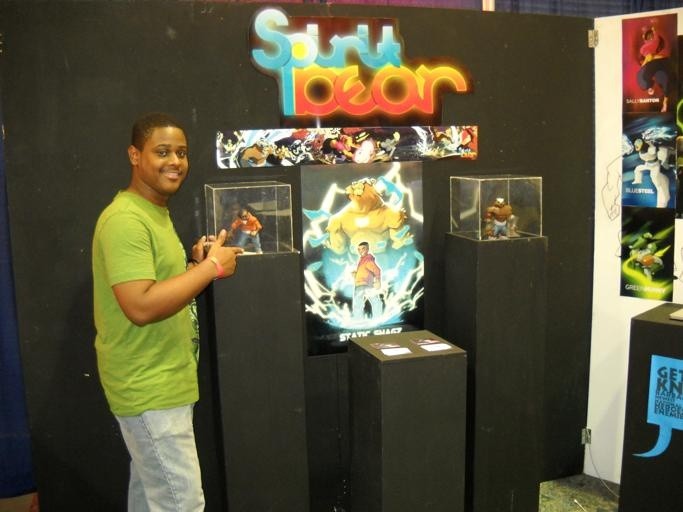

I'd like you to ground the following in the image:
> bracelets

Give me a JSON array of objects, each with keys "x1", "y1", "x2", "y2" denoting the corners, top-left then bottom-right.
[
  {"x1": 208, "y1": 256, "x2": 224, "y2": 280},
  {"x1": 191, "y1": 259, "x2": 201, "y2": 266}
]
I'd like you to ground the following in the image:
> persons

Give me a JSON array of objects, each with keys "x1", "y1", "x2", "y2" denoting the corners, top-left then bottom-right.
[
  {"x1": 223, "y1": 209, "x2": 265, "y2": 255},
  {"x1": 479, "y1": 197, "x2": 519, "y2": 240},
  {"x1": 348, "y1": 240, "x2": 383, "y2": 319},
  {"x1": 88, "y1": 110, "x2": 246, "y2": 512},
  {"x1": 216, "y1": 130, "x2": 402, "y2": 169},
  {"x1": 636, "y1": 24, "x2": 670, "y2": 113},
  {"x1": 629, "y1": 138, "x2": 670, "y2": 209}
]
